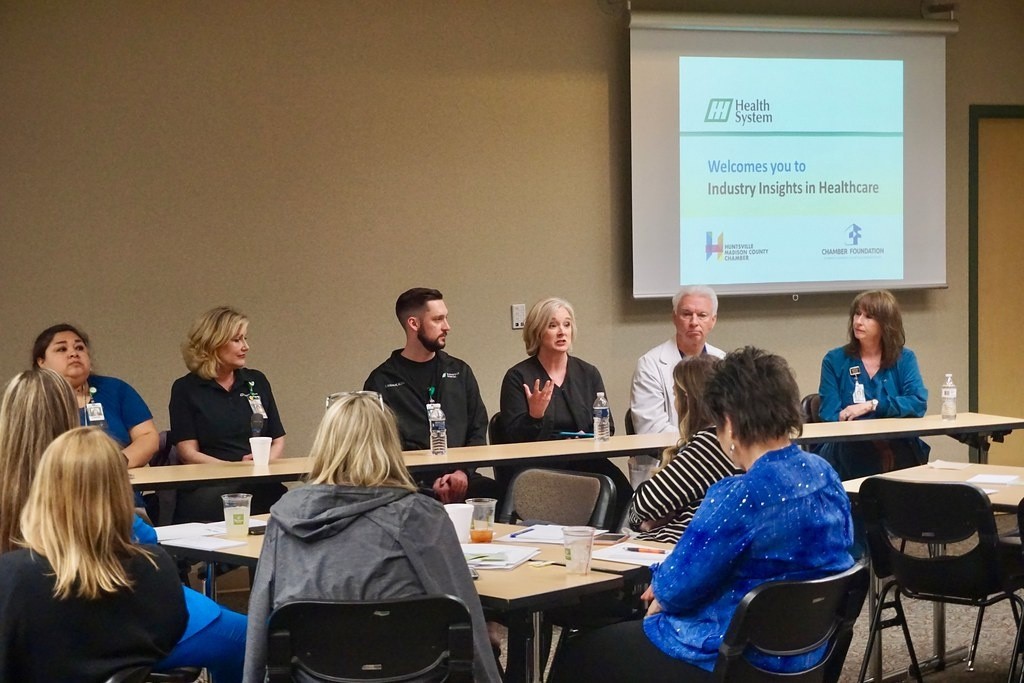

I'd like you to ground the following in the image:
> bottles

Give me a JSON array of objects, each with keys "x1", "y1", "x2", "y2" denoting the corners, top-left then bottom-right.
[
  {"x1": 430, "y1": 403, "x2": 447, "y2": 455},
  {"x1": 940, "y1": 374, "x2": 957, "y2": 421},
  {"x1": 592, "y1": 392, "x2": 610, "y2": 443}
]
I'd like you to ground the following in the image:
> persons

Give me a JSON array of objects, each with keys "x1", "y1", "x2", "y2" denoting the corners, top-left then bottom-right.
[
  {"x1": 0, "y1": 425, "x2": 189, "y2": 683},
  {"x1": 628, "y1": 353, "x2": 746, "y2": 542},
  {"x1": 813, "y1": 288, "x2": 932, "y2": 480},
  {"x1": 168, "y1": 306, "x2": 288, "y2": 525},
  {"x1": 500, "y1": 297, "x2": 635, "y2": 529},
  {"x1": 32, "y1": 323, "x2": 160, "y2": 507},
  {"x1": 552, "y1": 346, "x2": 855, "y2": 683},
  {"x1": 243, "y1": 392, "x2": 504, "y2": 683},
  {"x1": 631, "y1": 285, "x2": 728, "y2": 435},
  {"x1": 857, "y1": 391, "x2": 861, "y2": 397},
  {"x1": 362, "y1": 288, "x2": 496, "y2": 503},
  {"x1": 0, "y1": 365, "x2": 248, "y2": 683}
]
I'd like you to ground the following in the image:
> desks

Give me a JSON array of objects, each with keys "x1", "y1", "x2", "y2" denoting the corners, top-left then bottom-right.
[
  {"x1": 843, "y1": 460, "x2": 1024, "y2": 682},
  {"x1": 160, "y1": 512, "x2": 676, "y2": 683},
  {"x1": 129, "y1": 411, "x2": 1024, "y2": 489}
]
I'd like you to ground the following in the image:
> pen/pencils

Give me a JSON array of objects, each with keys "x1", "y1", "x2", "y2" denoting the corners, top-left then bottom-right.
[
  {"x1": 623, "y1": 547, "x2": 668, "y2": 554},
  {"x1": 509, "y1": 528, "x2": 534, "y2": 538}
]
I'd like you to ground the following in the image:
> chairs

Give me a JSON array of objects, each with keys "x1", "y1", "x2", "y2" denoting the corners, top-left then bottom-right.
[
  {"x1": 858, "y1": 475, "x2": 1024, "y2": 683},
  {"x1": 711, "y1": 565, "x2": 871, "y2": 683},
  {"x1": 499, "y1": 466, "x2": 616, "y2": 682},
  {"x1": 266, "y1": 594, "x2": 474, "y2": 683},
  {"x1": 799, "y1": 394, "x2": 822, "y2": 456}
]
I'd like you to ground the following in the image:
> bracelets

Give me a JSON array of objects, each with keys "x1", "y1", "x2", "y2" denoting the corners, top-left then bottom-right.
[{"x1": 872, "y1": 399, "x2": 878, "y2": 410}]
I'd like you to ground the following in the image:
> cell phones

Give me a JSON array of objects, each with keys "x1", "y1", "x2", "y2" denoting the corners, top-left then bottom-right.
[
  {"x1": 560, "y1": 431, "x2": 594, "y2": 438},
  {"x1": 593, "y1": 534, "x2": 629, "y2": 545},
  {"x1": 249, "y1": 526, "x2": 267, "y2": 535}
]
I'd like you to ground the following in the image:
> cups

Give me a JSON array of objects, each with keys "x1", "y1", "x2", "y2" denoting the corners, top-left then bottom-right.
[
  {"x1": 250, "y1": 436, "x2": 272, "y2": 465},
  {"x1": 444, "y1": 503, "x2": 474, "y2": 544},
  {"x1": 562, "y1": 526, "x2": 595, "y2": 576},
  {"x1": 465, "y1": 498, "x2": 497, "y2": 543},
  {"x1": 221, "y1": 494, "x2": 253, "y2": 541}
]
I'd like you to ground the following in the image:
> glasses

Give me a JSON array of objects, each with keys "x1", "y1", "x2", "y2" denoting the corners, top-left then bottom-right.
[{"x1": 325, "y1": 390, "x2": 384, "y2": 414}]
